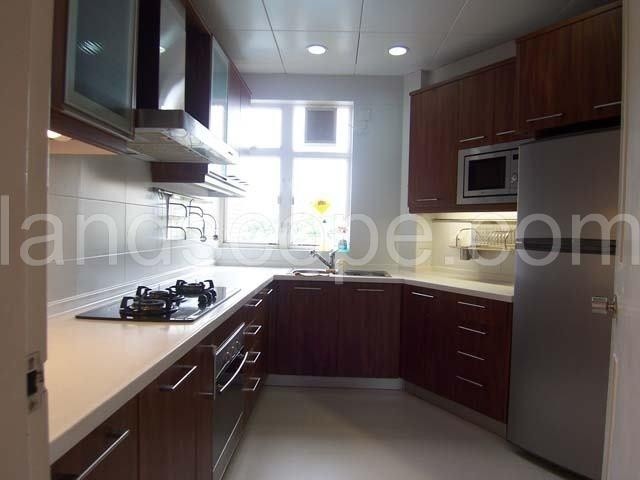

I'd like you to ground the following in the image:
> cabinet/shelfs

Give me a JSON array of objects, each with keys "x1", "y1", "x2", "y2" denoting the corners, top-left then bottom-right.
[
  {"x1": 50, "y1": 335, "x2": 213, "y2": 480},
  {"x1": 244, "y1": 282, "x2": 273, "y2": 404},
  {"x1": 407, "y1": 0, "x2": 622, "y2": 215},
  {"x1": 48, "y1": 0, "x2": 140, "y2": 158},
  {"x1": 152, "y1": 34, "x2": 252, "y2": 199},
  {"x1": 270, "y1": 281, "x2": 402, "y2": 378},
  {"x1": 402, "y1": 285, "x2": 513, "y2": 425}
]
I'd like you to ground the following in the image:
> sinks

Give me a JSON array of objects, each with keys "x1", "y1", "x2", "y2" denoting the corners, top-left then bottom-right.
[
  {"x1": 334, "y1": 268, "x2": 393, "y2": 278},
  {"x1": 290, "y1": 268, "x2": 345, "y2": 276},
  {"x1": 447, "y1": 244, "x2": 515, "y2": 276}
]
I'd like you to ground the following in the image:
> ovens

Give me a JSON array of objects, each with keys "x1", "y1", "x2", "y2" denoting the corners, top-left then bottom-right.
[
  {"x1": 463, "y1": 150, "x2": 518, "y2": 196},
  {"x1": 211, "y1": 323, "x2": 246, "y2": 469}
]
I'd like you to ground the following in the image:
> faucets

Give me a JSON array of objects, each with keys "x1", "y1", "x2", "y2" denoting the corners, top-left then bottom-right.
[
  {"x1": 455, "y1": 228, "x2": 482, "y2": 248},
  {"x1": 503, "y1": 228, "x2": 514, "y2": 250},
  {"x1": 311, "y1": 250, "x2": 339, "y2": 268}
]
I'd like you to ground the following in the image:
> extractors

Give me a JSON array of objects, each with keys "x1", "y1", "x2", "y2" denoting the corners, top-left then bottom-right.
[{"x1": 126, "y1": 4, "x2": 237, "y2": 163}]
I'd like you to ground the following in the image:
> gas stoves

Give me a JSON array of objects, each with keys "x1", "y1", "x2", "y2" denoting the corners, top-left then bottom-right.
[{"x1": 75, "y1": 279, "x2": 241, "y2": 323}]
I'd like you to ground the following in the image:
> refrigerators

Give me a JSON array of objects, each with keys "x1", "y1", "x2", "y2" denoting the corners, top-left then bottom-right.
[{"x1": 507, "y1": 129, "x2": 621, "y2": 480}]
